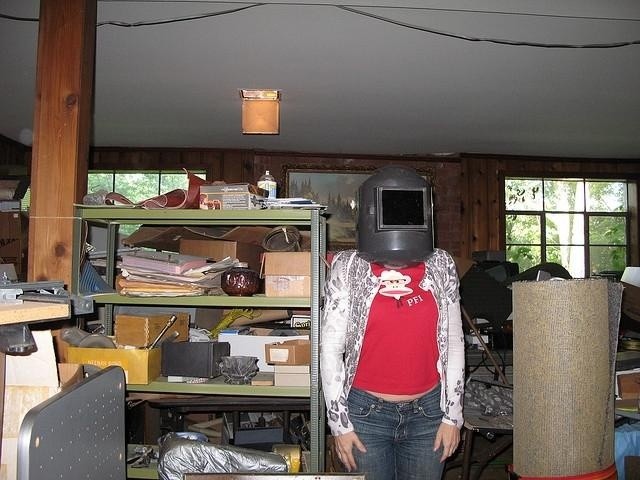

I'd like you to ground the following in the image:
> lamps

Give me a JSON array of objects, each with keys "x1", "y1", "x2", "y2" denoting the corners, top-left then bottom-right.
[{"x1": 239, "y1": 87, "x2": 281, "y2": 134}]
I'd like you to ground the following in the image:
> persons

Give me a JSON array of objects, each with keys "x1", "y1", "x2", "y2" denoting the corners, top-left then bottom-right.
[{"x1": 318, "y1": 249, "x2": 467, "y2": 479}]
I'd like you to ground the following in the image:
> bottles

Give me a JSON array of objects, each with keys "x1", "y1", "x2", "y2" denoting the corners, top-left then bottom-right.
[
  {"x1": 260, "y1": 171, "x2": 275, "y2": 201},
  {"x1": 220, "y1": 263, "x2": 260, "y2": 297}
]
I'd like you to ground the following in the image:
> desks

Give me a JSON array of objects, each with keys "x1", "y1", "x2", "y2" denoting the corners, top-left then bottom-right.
[{"x1": 148, "y1": 395, "x2": 311, "y2": 446}]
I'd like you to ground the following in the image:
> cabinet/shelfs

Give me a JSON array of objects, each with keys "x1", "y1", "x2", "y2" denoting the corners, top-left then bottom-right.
[{"x1": 74, "y1": 201, "x2": 329, "y2": 479}]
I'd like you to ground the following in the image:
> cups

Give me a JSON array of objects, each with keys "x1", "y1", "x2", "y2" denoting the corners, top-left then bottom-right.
[{"x1": 220, "y1": 356, "x2": 258, "y2": 385}]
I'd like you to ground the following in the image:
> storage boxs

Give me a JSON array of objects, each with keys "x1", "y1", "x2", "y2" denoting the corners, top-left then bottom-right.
[
  {"x1": 0, "y1": 353, "x2": 85, "y2": 480},
  {"x1": 175, "y1": 231, "x2": 312, "y2": 297},
  {"x1": 65, "y1": 310, "x2": 188, "y2": 385}
]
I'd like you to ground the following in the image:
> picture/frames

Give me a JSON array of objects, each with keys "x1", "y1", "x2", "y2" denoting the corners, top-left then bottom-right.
[{"x1": 281, "y1": 163, "x2": 434, "y2": 247}]
